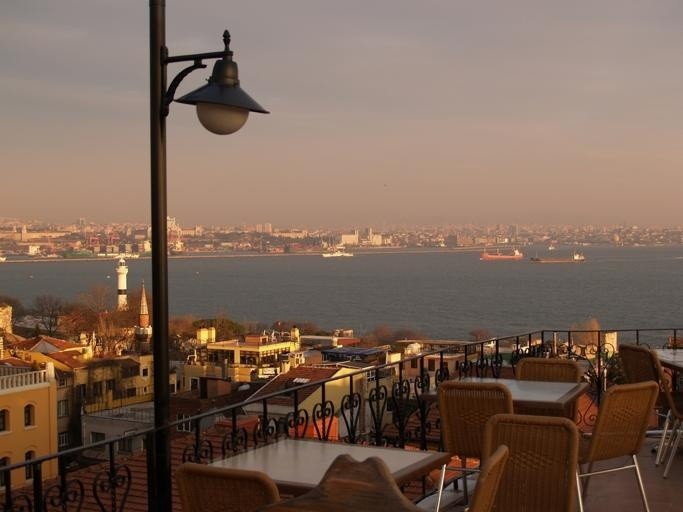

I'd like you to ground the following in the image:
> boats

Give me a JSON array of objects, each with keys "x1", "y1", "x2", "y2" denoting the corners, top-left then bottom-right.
[
  {"x1": 528, "y1": 248, "x2": 586, "y2": 264},
  {"x1": 545, "y1": 243, "x2": 556, "y2": 252},
  {"x1": 479, "y1": 245, "x2": 524, "y2": 261},
  {"x1": 321, "y1": 246, "x2": 354, "y2": 258}
]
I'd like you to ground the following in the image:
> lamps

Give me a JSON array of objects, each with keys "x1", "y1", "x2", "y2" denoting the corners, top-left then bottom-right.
[{"x1": 159, "y1": 28, "x2": 272, "y2": 135}]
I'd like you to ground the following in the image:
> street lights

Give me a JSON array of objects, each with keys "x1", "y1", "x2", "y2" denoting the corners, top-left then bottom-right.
[{"x1": 146, "y1": 0, "x2": 271, "y2": 511}]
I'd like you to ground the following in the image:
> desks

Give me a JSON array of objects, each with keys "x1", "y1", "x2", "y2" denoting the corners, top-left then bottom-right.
[
  {"x1": 649, "y1": 348, "x2": 682, "y2": 386},
  {"x1": 205, "y1": 437, "x2": 451, "y2": 491},
  {"x1": 420, "y1": 376, "x2": 590, "y2": 415}
]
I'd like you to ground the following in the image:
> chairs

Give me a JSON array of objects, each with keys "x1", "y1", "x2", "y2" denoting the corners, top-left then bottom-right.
[{"x1": 177, "y1": 333, "x2": 682, "y2": 511}]
